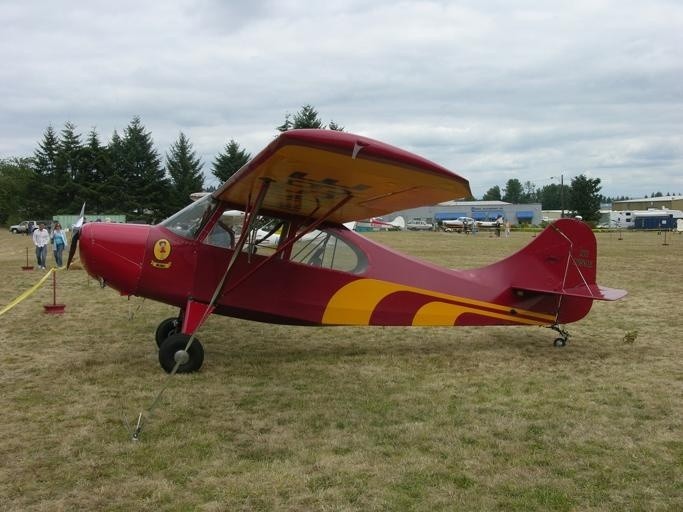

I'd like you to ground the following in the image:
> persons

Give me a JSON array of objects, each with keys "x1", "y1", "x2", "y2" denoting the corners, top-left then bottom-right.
[
  {"x1": 495, "y1": 221, "x2": 501, "y2": 228},
  {"x1": 24, "y1": 222, "x2": 32, "y2": 235},
  {"x1": 504, "y1": 219, "x2": 510, "y2": 235},
  {"x1": 33, "y1": 222, "x2": 68, "y2": 270},
  {"x1": 463, "y1": 218, "x2": 468, "y2": 233}
]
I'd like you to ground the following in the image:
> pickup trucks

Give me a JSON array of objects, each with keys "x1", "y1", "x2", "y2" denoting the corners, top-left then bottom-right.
[{"x1": 9, "y1": 221, "x2": 48, "y2": 234}]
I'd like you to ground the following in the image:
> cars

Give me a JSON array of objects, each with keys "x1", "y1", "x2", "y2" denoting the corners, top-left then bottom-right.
[{"x1": 407, "y1": 220, "x2": 433, "y2": 231}]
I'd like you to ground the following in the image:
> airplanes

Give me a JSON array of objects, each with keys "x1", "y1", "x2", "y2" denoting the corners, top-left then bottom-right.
[
  {"x1": 223, "y1": 209, "x2": 405, "y2": 250},
  {"x1": 441, "y1": 217, "x2": 503, "y2": 235},
  {"x1": 66, "y1": 128, "x2": 628, "y2": 375}
]
